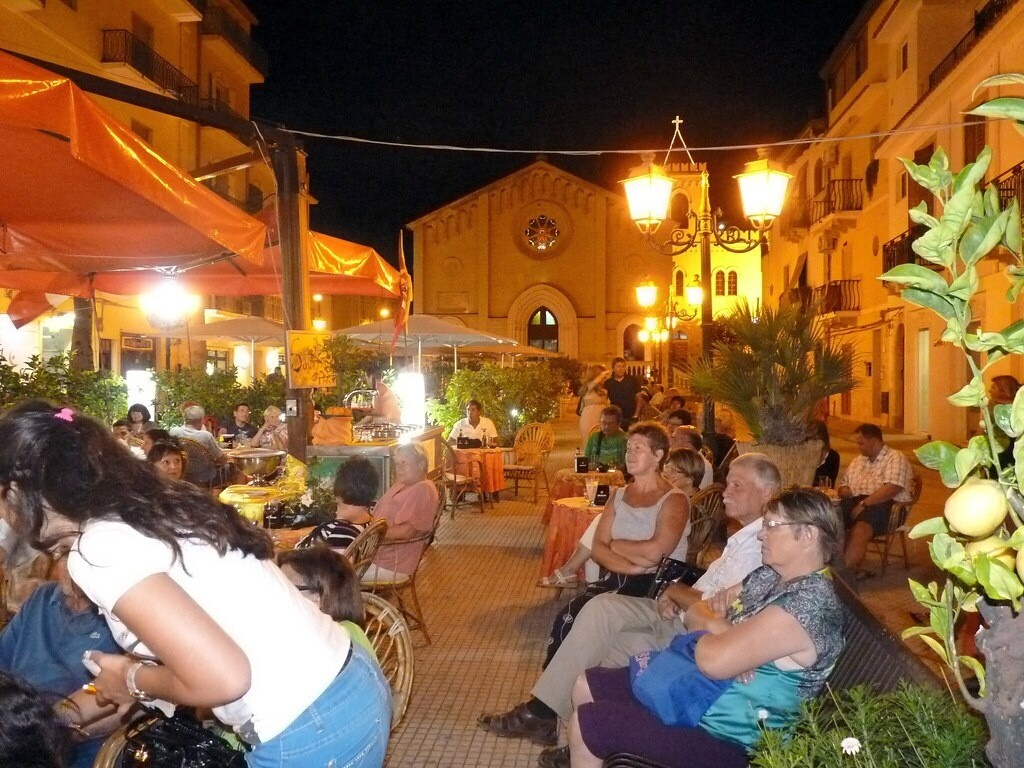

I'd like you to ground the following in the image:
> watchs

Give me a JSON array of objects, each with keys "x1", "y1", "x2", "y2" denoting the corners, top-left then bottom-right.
[
  {"x1": 859, "y1": 500, "x2": 868, "y2": 509},
  {"x1": 127, "y1": 660, "x2": 159, "y2": 701}
]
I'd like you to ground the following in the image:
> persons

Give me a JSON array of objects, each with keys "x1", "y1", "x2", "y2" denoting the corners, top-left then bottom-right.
[
  {"x1": 538, "y1": 484, "x2": 845, "y2": 768},
  {"x1": 446, "y1": 396, "x2": 501, "y2": 453},
  {"x1": 0, "y1": 399, "x2": 440, "y2": 768},
  {"x1": 542, "y1": 421, "x2": 692, "y2": 664},
  {"x1": 808, "y1": 413, "x2": 842, "y2": 489},
  {"x1": 358, "y1": 364, "x2": 400, "y2": 428},
  {"x1": 535, "y1": 355, "x2": 716, "y2": 589},
  {"x1": 473, "y1": 450, "x2": 787, "y2": 768},
  {"x1": 838, "y1": 425, "x2": 915, "y2": 580}
]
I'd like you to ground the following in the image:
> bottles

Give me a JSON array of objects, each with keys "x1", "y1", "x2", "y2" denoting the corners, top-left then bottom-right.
[
  {"x1": 458, "y1": 428, "x2": 463, "y2": 437},
  {"x1": 480, "y1": 427, "x2": 488, "y2": 447},
  {"x1": 574, "y1": 447, "x2": 581, "y2": 473}
]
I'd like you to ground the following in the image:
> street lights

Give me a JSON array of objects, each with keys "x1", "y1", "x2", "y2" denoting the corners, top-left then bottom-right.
[
  {"x1": 617, "y1": 148, "x2": 793, "y2": 461},
  {"x1": 635, "y1": 273, "x2": 705, "y2": 389}
]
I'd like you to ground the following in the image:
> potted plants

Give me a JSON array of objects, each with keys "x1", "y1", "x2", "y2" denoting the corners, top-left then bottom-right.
[{"x1": 665, "y1": 287, "x2": 871, "y2": 489}]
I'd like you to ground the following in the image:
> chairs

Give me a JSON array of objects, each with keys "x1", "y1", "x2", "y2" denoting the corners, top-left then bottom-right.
[
  {"x1": 341, "y1": 518, "x2": 414, "y2": 734},
  {"x1": 435, "y1": 435, "x2": 486, "y2": 518},
  {"x1": 499, "y1": 422, "x2": 554, "y2": 504},
  {"x1": 867, "y1": 466, "x2": 922, "y2": 573},
  {"x1": 174, "y1": 435, "x2": 236, "y2": 495},
  {"x1": 356, "y1": 467, "x2": 447, "y2": 646},
  {"x1": 689, "y1": 483, "x2": 726, "y2": 569}
]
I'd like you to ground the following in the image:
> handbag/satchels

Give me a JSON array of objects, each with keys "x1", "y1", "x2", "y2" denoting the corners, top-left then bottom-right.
[
  {"x1": 121, "y1": 705, "x2": 248, "y2": 768},
  {"x1": 628, "y1": 629, "x2": 735, "y2": 727}
]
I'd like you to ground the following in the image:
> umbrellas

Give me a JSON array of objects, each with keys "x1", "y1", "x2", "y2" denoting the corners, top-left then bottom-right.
[
  {"x1": 329, "y1": 311, "x2": 565, "y2": 396},
  {"x1": 135, "y1": 312, "x2": 288, "y2": 383}
]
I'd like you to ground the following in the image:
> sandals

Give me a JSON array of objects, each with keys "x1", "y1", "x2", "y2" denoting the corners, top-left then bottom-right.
[
  {"x1": 538, "y1": 745, "x2": 570, "y2": 768},
  {"x1": 537, "y1": 569, "x2": 579, "y2": 590},
  {"x1": 476, "y1": 703, "x2": 557, "y2": 746}
]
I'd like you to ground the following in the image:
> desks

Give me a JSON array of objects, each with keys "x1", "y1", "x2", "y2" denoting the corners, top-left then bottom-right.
[
  {"x1": 539, "y1": 468, "x2": 625, "y2": 527},
  {"x1": 536, "y1": 494, "x2": 604, "y2": 603},
  {"x1": 452, "y1": 444, "x2": 508, "y2": 507}
]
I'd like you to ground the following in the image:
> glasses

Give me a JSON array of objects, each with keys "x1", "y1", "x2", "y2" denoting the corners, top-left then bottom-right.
[{"x1": 762, "y1": 519, "x2": 814, "y2": 530}]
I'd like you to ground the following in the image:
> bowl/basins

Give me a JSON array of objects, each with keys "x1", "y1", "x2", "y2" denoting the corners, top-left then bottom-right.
[
  {"x1": 228, "y1": 450, "x2": 284, "y2": 486},
  {"x1": 353, "y1": 421, "x2": 422, "y2": 443}
]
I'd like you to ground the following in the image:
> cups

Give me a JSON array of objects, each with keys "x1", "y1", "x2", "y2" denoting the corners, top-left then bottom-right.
[
  {"x1": 819, "y1": 476, "x2": 831, "y2": 493},
  {"x1": 608, "y1": 461, "x2": 616, "y2": 472},
  {"x1": 590, "y1": 455, "x2": 599, "y2": 471},
  {"x1": 583, "y1": 489, "x2": 590, "y2": 502},
  {"x1": 488, "y1": 435, "x2": 498, "y2": 448},
  {"x1": 585, "y1": 478, "x2": 599, "y2": 507}
]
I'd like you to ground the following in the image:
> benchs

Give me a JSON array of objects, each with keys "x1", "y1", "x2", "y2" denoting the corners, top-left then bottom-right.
[{"x1": 601, "y1": 565, "x2": 992, "y2": 768}]
